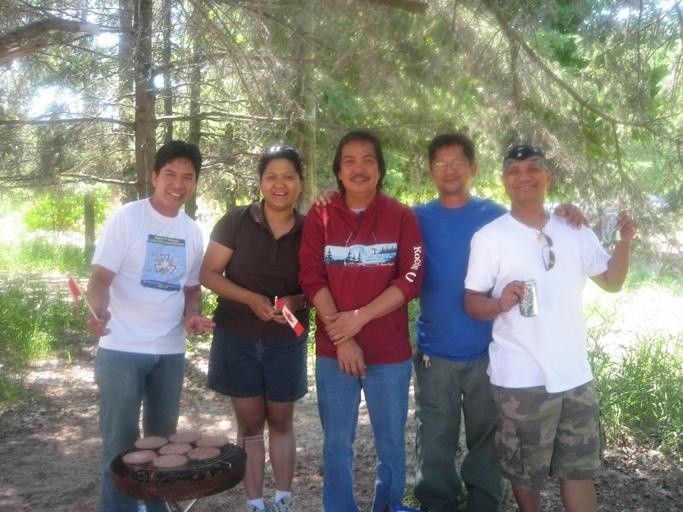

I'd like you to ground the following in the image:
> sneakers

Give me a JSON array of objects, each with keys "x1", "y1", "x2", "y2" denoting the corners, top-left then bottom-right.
[
  {"x1": 372, "y1": 499, "x2": 422, "y2": 512},
  {"x1": 246, "y1": 497, "x2": 297, "y2": 512}
]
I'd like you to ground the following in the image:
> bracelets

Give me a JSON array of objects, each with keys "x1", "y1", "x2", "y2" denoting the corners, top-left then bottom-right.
[{"x1": 299, "y1": 294, "x2": 306, "y2": 309}]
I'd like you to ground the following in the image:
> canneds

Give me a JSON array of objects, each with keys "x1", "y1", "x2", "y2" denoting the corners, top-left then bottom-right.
[
  {"x1": 602, "y1": 207, "x2": 620, "y2": 243},
  {"x1": 518, "y1": 281, "x2": 537, "y2": 317}
]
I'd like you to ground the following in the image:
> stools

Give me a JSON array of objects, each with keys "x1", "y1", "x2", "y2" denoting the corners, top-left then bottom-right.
[{"x1": 110, "y1": 437, "x2": 247, "y2": 512}]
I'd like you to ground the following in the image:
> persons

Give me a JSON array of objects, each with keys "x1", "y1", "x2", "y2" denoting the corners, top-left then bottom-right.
[
  {"x1": 310, "y1": 131, "x2": 589, "y2": 512},
  {"x1": 84, "y1": 140, "x2": 221, "y2": 512},
  {"x1": 295, "y1": 130, "x2": 426, "y2": 512},
  {"x1": 459, "y1": 139, "x2": 638, "y2": 512},
  {"x1": 199, "y1": 147, "x2": 314, "y2": 512}
]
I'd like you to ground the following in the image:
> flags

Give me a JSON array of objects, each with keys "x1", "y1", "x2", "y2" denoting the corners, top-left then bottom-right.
[
  {"x1": 273, "y1": 296, "x2": 305, "y2": 338},
  {"x1": 68, "y1": 276, "x2": 82, "y2": 304}
]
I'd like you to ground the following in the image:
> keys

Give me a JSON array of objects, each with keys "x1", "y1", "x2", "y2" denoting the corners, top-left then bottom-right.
[{"x1": 421, "y1": 352, "x2": 431, "y2": 370}]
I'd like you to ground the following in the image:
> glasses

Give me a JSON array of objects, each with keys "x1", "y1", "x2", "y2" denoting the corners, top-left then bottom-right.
[{"x1": 431, "y1": 160, "x2": 472, "y2": 170}]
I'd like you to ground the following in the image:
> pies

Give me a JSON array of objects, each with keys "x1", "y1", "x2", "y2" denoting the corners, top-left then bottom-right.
[{"x1": 122, "y1": 431, "x2": 228, "y2": 468}]
